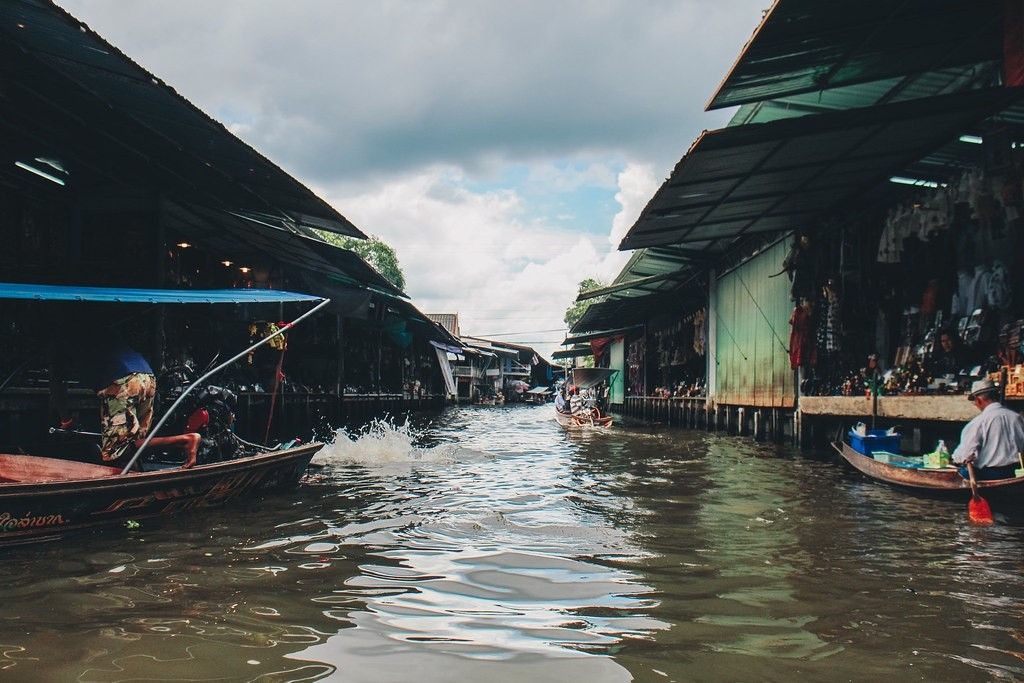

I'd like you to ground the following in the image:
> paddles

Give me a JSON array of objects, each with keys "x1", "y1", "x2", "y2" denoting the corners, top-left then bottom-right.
[{"x1": 966, "y1": 461, "x2": 995, "y2": 525}]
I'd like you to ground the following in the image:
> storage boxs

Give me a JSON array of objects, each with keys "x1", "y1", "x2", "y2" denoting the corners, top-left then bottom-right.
[{"x1": 848, "y1": 428, "x2": 949, "y2": 469}]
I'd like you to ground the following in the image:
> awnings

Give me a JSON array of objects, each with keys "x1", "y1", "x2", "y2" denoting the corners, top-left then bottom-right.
[
  {"x1": 705, "y1": 0, "x2": 1024, "y2": 110},
  {"x1": 617, "y1": 84, "x2": 1024, "y2": 251},
  {"x1": 431, "y1": 340, "x2": 519, "y2": 363},
  {"x1": 604, "y1": 245, "x2": 720, "y2": 302},
  {"x1": 569, "y1": 292, "x2": 678, "y2": 336},
  {"x1": 561, "y1": 326, "x2": 643, "y2": 346},
  {"x1": 0, "y1": 0, "x2": 370, "y2": 240},
  {"x1": 551, "y1": 347, "x2": 594, "y2": 360},
  {"x1": 174, "y1": 200, "x2": 408, "y2": 297},
  {"x1": 291, "y1": 267, "x2": 466, "y2": 347}
]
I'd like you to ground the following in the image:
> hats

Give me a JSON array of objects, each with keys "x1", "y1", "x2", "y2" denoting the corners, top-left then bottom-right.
[{"x1": 968, "y1": 381, "x2": 1001, "y2": 401}]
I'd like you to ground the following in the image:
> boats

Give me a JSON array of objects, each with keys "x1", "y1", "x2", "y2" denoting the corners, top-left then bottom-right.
[
  {"x1": 1, "y1": 281, "x2": 331, "y2": 548},
  {"x1": 526, "y1": 385, "x2": 554, "y2": 406},
  {"x1": 555, "y1": 331, "x2": 620, "y2": 438},
  {"x1": 830, "y1": 421, "x2": 1023, "y2": 500}
]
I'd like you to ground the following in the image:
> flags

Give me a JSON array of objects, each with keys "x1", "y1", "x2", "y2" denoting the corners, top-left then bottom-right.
[{"x1": 532, "y1": 353, "x2": 539, "y2": 366}]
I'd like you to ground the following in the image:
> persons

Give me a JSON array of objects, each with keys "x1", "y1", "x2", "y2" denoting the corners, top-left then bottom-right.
[
  {"x1": 789, "y1": 299, "x2": 818, "y2": 371},
  {"x1": 905, "y1": 355, "x2": 917, "y2": 369},
  {"x1": 816, "y1": 283, "x2": 843, "y2": 376},
  {"x1": 51, "y1": 312, "x2": 200, "y2": 470},
  {"x1": 789, "y1": 235, "x2": 821, "y2": 303},
  {"x1": 869, "y1": 354, "x2": 882, "y2": 379},
  {"x1": 937, "y1": 328, "x2": 979, "y2": 377},
  {"x1": 952, "y1": 379, "x2": 1024, "y2": 483}
]
